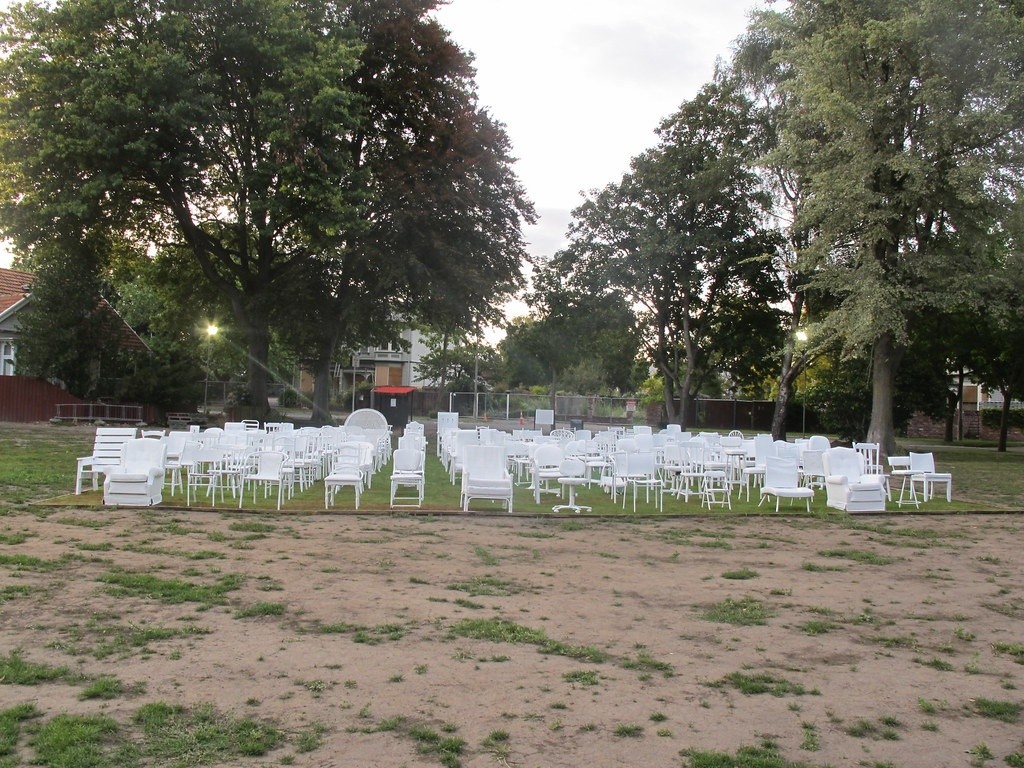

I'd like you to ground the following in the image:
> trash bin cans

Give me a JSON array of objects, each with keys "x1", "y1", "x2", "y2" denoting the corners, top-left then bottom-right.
[{"x1": 354, "y1": 389, "x2": 408, "y2": 430}]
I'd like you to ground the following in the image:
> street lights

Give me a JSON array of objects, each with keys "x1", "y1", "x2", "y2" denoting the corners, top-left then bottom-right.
[
  {"x1": 202, "y1": 325, "x2": 218, "y2": 414},
  {"x1": 794, "y1": 331, "x2": 807, "y2": 438}
]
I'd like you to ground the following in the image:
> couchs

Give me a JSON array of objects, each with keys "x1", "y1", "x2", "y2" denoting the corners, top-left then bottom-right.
[
  {"x1": 104, "y1": 437, "x2": 167, "y2": 507},
  {"x1": 822, "y1": 446, "x2": 886, "y2": 513}
]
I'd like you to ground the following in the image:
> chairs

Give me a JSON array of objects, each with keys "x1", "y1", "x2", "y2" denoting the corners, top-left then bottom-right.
[{"x1": 75, "y1": 409, "x2": 953, "y2": 514}]
